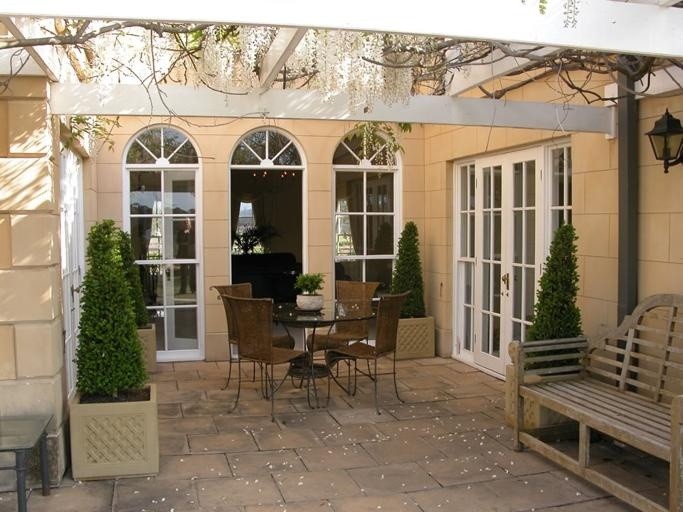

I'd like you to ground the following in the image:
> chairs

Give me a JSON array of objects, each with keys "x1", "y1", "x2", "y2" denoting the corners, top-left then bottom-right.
[
  {"x1": 325, "y1": 289, "x2": 411, "y2": 415},
  {"x1": 307, "y1": 279, "x2": 379, "y2": 395},
  {"x1": 209, "y1": 283, "x2": 295, "y2": 391},
  {"x1": 216, "y1": 293, "x2": 314, "y2": 425}
]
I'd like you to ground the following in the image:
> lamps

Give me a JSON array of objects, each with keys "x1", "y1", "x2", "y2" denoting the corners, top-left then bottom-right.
[{"x1": 643, "y1": 107, "x2": 683, "y2": 173}]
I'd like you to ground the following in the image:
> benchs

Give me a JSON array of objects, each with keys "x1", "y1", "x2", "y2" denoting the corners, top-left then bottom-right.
[{"x1": 508, "y1": 292, "x2": 683, "y2": 512}]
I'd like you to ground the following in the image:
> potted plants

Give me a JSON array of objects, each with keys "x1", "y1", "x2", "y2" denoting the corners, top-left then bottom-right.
[
  {"x1": 64, "y1": 222, "x2": 160, "y2": 481},
  {"x1": 118, "y1": 230, "x2": 157, "y2": 375},
  {"x1": 388, "y1": 221, "x2": 436, "y2": 360},
  {"x1": 505, "y1": 225, "x2": 593, "y2": 442},
  {"x1": 292, "y1": 272, "x2": 326, "y2": 310}
]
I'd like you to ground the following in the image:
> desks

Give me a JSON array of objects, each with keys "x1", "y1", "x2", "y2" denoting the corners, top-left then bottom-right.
[
  {"x1": 0, "y1": 413, "x2": 55, "y2": 512},
  {"x1": 272, "y1": 303, "x2": 377, "y2": 380}
]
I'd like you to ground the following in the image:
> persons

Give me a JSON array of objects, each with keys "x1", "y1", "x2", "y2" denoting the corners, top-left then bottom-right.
[{"x1": 171, "y1": 214, "x2": 197, "y2": 297}]
